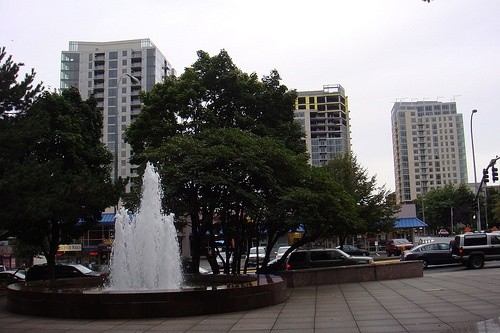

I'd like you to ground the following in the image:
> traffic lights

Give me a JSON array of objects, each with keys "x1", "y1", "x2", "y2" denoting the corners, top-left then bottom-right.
[
  {"x1": 483, "y1": 169, "x2": 489, "y2": 182},
  {"x1": 492, "y1": 167, "x2": 499, "y2": 183}
]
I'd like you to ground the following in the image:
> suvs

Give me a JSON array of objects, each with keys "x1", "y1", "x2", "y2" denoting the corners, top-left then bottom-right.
[
  {"x1": 449, "y1": 232, "x2": 500, "y2": 269},
  {"x1": 284, "y1": 247, "x2": 374, "y2": 271},
  {"x1": 24, "y1": 262, "x2": 103, "y2": 280},
  {"x1": 385, "y1": 238, "x2": 414, "y2": 257}
]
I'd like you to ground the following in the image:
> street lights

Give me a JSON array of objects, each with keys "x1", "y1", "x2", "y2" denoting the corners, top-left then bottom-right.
[
  {"x1": 113, "y1": 73, "x2": 141, "y2": 218},
  {"x1": 470, "y1": 109, "x2": 483, "y2": 230}
]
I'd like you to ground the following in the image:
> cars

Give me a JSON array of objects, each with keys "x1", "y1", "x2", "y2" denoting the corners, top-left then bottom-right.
[
  {"x1": 336, "y1": 245, "x2": 371, "y2": 256},
  {"x1": 275, "y1": 245, "x2": 295, "y2": 261},
  {"x1": 400, "y1": 241, "x2": 453, "y2": 268},
  {"x1": 0, "y1": 270, "x2": 25, "y2": 286},
  {"x1": 244, "y1": 246, "x2": 270, "y2": 267},
  {"x1": 216, "y1": 251, "x2": 234, "y2": 269},
  {"x1": 438, "y1": 229, "x2": 449, "y2": 237}
]
